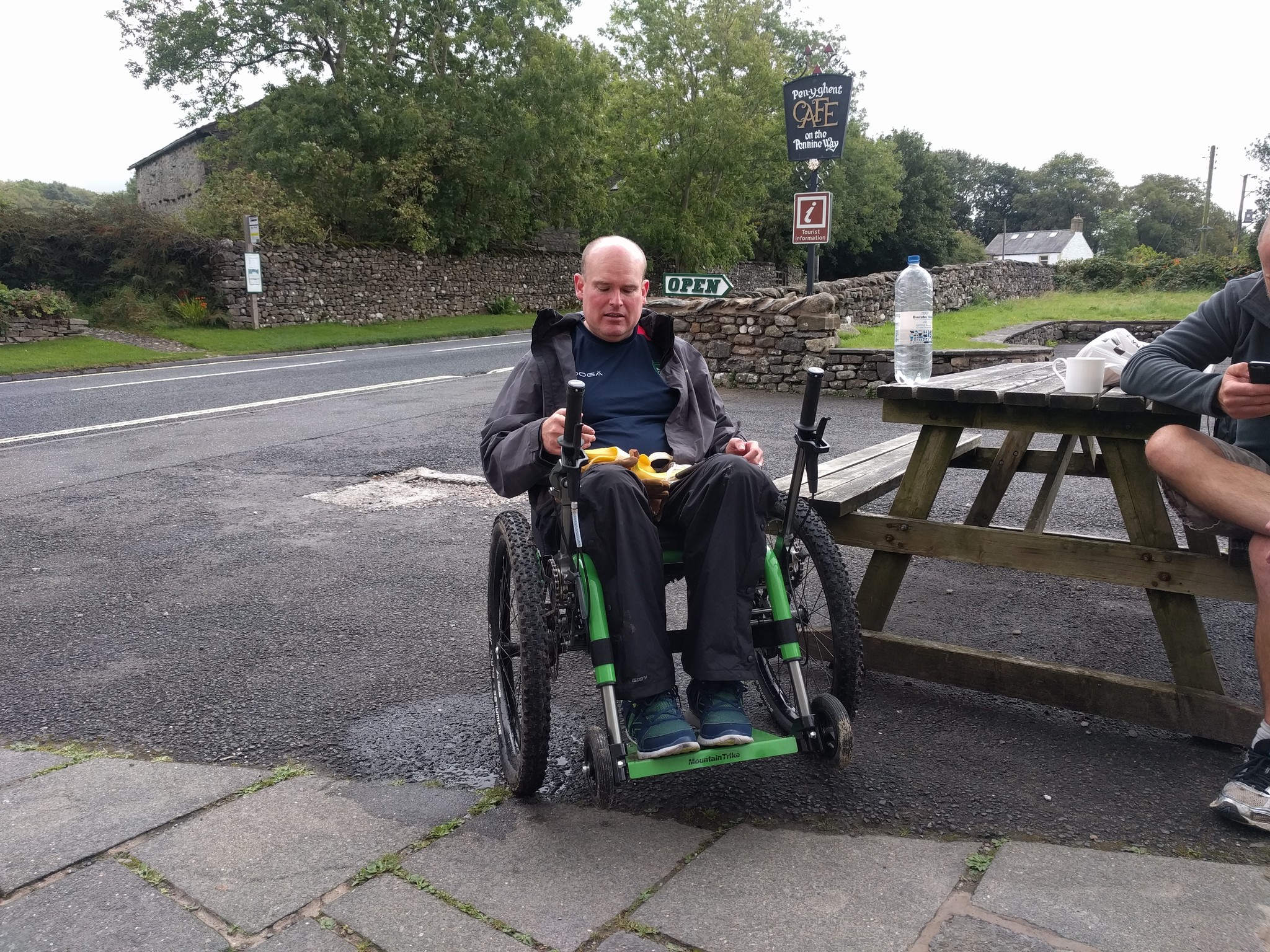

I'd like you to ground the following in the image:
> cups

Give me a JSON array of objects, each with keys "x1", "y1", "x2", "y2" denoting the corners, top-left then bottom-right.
[{"x1": 1052, "y1": 357, "x2": 1106, "y2": 394}]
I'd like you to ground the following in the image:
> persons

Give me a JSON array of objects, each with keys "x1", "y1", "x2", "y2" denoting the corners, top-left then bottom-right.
[
  {"x1": 1119, "y1": 214, "x2": 1269, "y2": 833},
  {"x1": 480, "y1": 238, "x2": 782, "y2": 760}
]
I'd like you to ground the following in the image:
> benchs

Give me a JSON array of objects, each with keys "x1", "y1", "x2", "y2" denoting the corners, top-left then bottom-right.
[{"x1": 771, "y1": 431, "x2": 983, "y2": 518}]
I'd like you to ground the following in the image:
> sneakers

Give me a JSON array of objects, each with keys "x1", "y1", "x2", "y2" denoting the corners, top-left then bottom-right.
[
  {"x1": 619, "y1": 684, "x2": 701, "y2": 760},
  {"x1": 683, "y1": 681, "x2": 755, "y2": 746},
  {"x1": 1207, "y1": 740, "x2": 1270, "y2": 833}
]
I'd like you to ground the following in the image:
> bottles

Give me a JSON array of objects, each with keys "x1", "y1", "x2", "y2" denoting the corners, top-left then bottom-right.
[{"x1": 895, "y1": 255, "x2": 933, "y2": 385}]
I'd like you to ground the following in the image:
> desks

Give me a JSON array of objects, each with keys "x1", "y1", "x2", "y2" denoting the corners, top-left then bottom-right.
[{"x1": 796, "y1": 361, "x2": 1263, "y2": 747}]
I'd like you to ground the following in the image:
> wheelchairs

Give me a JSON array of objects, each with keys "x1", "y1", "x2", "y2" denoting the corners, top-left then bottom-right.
[{"x1": 485, "y1": 365, "x2": 868, "y2": 813}]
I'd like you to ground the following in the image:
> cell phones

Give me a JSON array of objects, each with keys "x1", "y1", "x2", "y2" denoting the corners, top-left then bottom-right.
[{"x1": 1248, "y1": 361, "x2": 1270, "y2": 384}]
